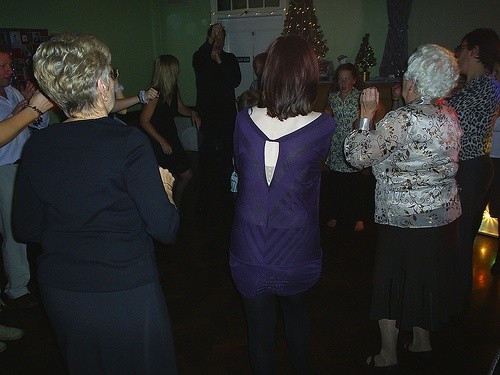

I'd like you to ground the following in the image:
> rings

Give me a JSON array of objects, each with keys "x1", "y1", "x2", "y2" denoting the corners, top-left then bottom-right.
[{"x1": 362, "y1": 93, "x2": 366, "y2": 95}]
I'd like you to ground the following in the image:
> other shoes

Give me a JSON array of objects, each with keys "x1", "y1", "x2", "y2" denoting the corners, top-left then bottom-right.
[
  {"x1": 0, "y1": 326, "x2": 24, "y2": 352},
  {"x1": 1, "y1": 293, "x2": 38, "y2": 312},
  {"x1": 399, "y1": 342, "x2": 435, "y2": 357},
  {"x1": 361, "y1": 351, "x2": 397, "y2": 369}
]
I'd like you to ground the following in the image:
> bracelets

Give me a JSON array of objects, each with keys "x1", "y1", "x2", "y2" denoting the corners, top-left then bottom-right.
[
  {"x1": 164, "y1": 182, "x2": 173, "y2": 191},
  {"x1": 354, "y1": 117, "x2": 374, "y2": 131},
  {"x1": 114, "y1": 86, "x2": 124, "y2": 93},
  {"x1": 139, "y1": 90, "x2": 148, "y2": 104},
  {"x1": 26, "y1": 105, "x2": 43, "y2": 122}
]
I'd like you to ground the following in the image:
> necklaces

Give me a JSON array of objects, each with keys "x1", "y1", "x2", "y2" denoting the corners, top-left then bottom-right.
[{"x1": 337, "y1": 91, "x2": 349, "y2": 104}]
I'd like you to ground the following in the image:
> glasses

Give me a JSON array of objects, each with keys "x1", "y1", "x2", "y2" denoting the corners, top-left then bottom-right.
[
  {"x1": 455, "y1": 40, "x2": 472, "y2": 50},
  {"x1": 109, "y1": 68, "x2": 119, "y2": 80}
]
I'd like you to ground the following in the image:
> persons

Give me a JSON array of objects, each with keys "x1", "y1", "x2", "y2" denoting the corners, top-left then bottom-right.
[
  {"x1": 1, "y1": 24, "x2": 499, "y2": 374},
  {"x1": 234, "y1": 35, "x2": 334, "y2": 375},
  {"x1": 10, "y1": 32, "x2": 178, "y2": 375},
  {"x1": 12, "y1": 34, "x2": 20, "y2": 47},
  {"x1": 33, "y1": 33, "x2": 40, "y2": 43}
]
